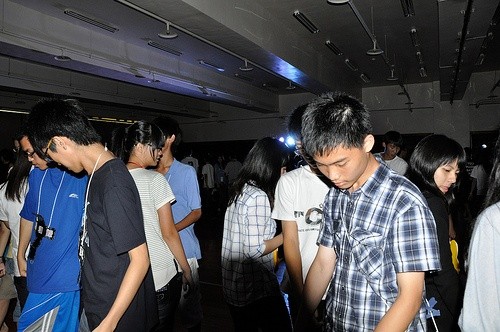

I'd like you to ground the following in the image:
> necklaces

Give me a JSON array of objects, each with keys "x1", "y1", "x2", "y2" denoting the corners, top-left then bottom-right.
[{"x1": 128, "y1": 161, "x2": 142, "y2": 168}]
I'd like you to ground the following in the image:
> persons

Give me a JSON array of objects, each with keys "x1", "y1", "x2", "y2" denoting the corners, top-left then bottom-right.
[{"x1": 0, "y1": 90, "x2": 500, "y2": 332}]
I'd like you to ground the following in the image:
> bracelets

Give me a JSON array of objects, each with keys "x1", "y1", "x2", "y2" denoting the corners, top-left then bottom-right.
[{"x1": 0, "y1": 257, "x2": 4, "y2": 263}]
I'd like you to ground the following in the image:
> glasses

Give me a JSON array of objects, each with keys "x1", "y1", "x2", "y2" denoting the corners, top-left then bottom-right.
[
  {"x1": 293, "y1": 148, "x2": 306, "y2": 156},
  {"x1": 41, "y1": 138, "x2": 52, "y2": 163},
  {"x1": 24, "y1": 150, "x2": 36, "y2": 158},
  {"x1": 133, "y1": 142, "x2": 163, "y2": 153}
]
